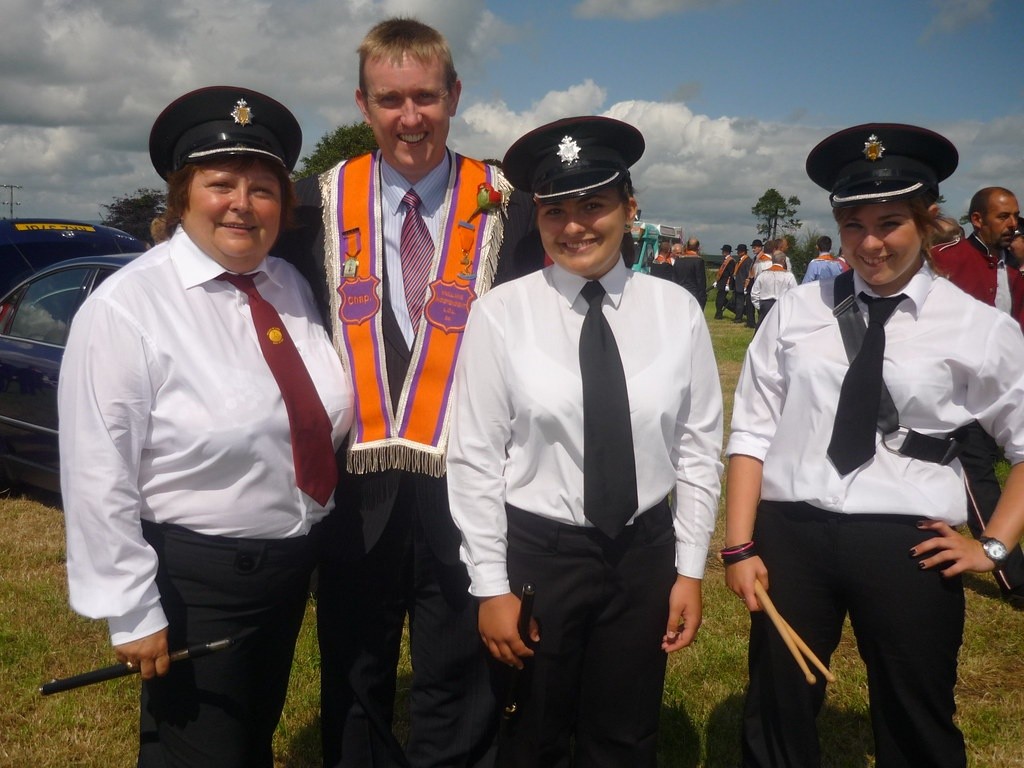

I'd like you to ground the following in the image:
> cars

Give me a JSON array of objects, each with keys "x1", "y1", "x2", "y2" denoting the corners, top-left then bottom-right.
[{"x1": 0, "y1": 216, "x2": 162, "y2": 500}]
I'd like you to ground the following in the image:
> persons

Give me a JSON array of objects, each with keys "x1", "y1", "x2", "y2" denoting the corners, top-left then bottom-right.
[
  {"x1": 743, "y1": 239, "x2": 764, "y2": 327},
  {"x1": 650, "y1": 242, "x2": 680, "y2": 284},
  {"x1": 753, "y1": 241, "x2": 777, "y2": 283},
  {"x1": 802, "y1": 235, "x2": 844, "y2": 286},
  {"x1": 56, "y1": 84, "x2": 355, "y2": 768},
  {"x1": 711, "y1": 244, "x2": 737, "y2": 320},
  {"x1": 729, "y1": 243, "x2": 752, "y2": 323},
  {"x1": 931, "y1": 185, "x2": 1024, "y2": 612},
  {"x1": 666, "y1": 243, "x2": 684, "y2": 266},
  {"x1": 446, "y1": 115, "x2": 725, "y2": 768},
  {"x1": 835, "y1": 246, "x2": 851, "y2": 271},
  {"x1": 270, "y1": 12, "x2": 636, "y2": 768},
  {"x1": 774, "y1": 235, "x2": 794, "y2": 273},
  {"x1": 673, "y1": 237, "x2": 708, "y2": 313},
  {"x1": 725, "y1": 121, "x2": 1024, "y2": 768},
  {"x1": 750, "y1": 251, "x2": 798, "y2": 339}
]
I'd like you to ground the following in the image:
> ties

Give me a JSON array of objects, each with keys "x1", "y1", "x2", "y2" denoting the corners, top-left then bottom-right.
[
  {"x1": 828, "y1": 291, "x2": 907, "y2": 476},
  {"x1": 580, "y1": 280, "x2": 640, "y2": 541},
  {"x1": 216, "y1": 269, "x2": 339, "y2": 506},
  {"x1": 399, "y1": 187, "x2": 436, "y2": 336}
]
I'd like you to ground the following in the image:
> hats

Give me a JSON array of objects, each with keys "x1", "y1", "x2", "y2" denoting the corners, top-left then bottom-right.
[
  {"x1": 720, "y1": 244, "x2": 733, "y2": 252},
  {"x1": 750, "y1": 239, "x2": 764, "y2": 247},
  {"x1": 501, "y1": 115, "x2": 647, "y2": 204},
  {"x1": 149, "y1": 86, "x2": 303, "y2": 175},
  {"x1": 805, "y1": 121, "x2": 959, "y2": 206},
  {"x1": 736, "y1": 243, "x2": 749, "y2": 251}
]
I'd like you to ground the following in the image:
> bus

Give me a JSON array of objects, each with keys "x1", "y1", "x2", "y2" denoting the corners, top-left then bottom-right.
[{"x1": 632, "y1": 220, "x2": 684, "y2": 279}]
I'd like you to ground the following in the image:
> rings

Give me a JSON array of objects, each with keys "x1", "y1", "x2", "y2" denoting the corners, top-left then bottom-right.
[{"x1": 126, "y1": 662, "x2": 140, "y2": 670}]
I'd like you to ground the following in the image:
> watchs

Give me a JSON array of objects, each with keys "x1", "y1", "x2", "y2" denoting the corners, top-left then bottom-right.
[{"x1": 980, "y1": 535, "x2": 1007, "y2": 571}]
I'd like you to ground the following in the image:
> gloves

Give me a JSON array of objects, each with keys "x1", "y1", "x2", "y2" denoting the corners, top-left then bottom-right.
[
  {"x1": 744, "y1": 288, "x2": 748, "y2": 295},
  {"x1": 725, "y1": 286, "x2": 729, "y2": 292},
  {"x1": 713, "y1": 282, "x2": 717, "y2": 288}
]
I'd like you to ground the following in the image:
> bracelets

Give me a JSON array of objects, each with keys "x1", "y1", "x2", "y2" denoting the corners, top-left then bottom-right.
[{"x1": 717, "y1": 542, "x2": 760, "y2": 567}]
